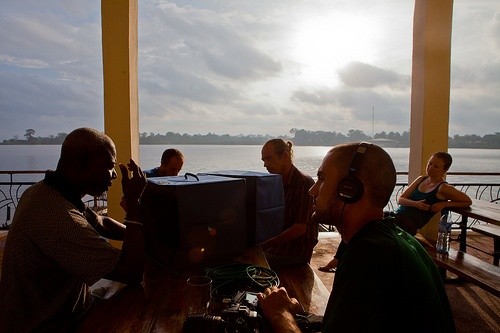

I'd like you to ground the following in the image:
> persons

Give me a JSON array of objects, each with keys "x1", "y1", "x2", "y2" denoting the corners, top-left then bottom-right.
[
  {"x1": 256, "y1": 138, "x2": 319, "y2": 274},
  {"x1": 0, "y1": 127, "x2": 146, "y2": 333},
  {"x1": 119, "y1": 148, "x2": 183, "y2": 213},
  {"x1": 317, "y1": 151, "x2": 472, "y2": 273},
  {"x1": 255, "y1": 141, "x2": 455, "y2": 333}
]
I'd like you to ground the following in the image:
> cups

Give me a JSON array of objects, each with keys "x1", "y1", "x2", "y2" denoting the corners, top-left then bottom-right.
[{"x1": 186, "y1": 275, "x2": 212, "y2": 316}]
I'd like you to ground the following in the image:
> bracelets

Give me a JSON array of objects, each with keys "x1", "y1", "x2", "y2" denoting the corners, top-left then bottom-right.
[{"x1": 123, "y1": 220, "x2": 144, "y2": 226}]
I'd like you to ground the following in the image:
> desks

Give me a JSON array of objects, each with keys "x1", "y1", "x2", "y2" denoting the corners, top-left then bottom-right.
[
  {"x1": 439, "y1": 198, "x2": 500, "y2": 253},
  {"x1": 74, "y1": 245, "x2": 277, "y2": 333}
]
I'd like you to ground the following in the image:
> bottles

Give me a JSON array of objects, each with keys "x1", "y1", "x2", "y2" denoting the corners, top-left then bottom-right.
[{"x1": 435, "y1": 211, "x2": 451, "y2": 253}]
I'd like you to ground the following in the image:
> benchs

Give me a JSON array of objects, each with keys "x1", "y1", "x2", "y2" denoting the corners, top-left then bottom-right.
[
  {"x1": 470, "y1": 224, "x2": 500, "y2": 266},
  {"x1": 421, "y1": 243, "x2": 500, "y2": 298}
]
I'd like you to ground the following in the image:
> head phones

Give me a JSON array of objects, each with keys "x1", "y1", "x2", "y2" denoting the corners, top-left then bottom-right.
[{"x1": 336, "y1": 142, "x2": 369, "y2": 204}]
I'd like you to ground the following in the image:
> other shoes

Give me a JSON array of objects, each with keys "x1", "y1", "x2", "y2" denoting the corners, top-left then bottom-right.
[{"x1": 318, "y1": 264, "x2": 337, "y2": 272}]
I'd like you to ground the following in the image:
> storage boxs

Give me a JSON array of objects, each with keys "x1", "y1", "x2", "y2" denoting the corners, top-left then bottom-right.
[
  {"x1": 141, "y1": 170, "x2": 247, "y2": 260},
  {"x1": 209, "y1": 173, "x2": 285, "y2": 248}
]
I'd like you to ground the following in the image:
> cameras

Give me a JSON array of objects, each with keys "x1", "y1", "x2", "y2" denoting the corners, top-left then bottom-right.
[{"x1": 182, "y1": 303, "x2": 263, "y2": 333}]
